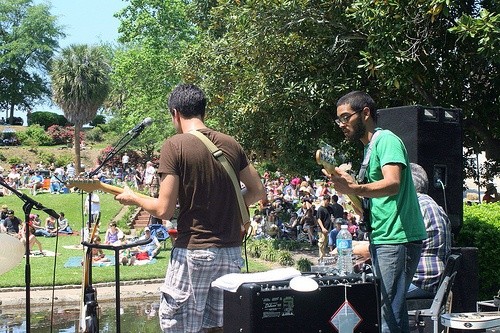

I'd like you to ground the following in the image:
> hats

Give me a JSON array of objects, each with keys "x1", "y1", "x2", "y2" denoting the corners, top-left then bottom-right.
[
  {"x1": 30, "y1": 214, "x2": 37, "y2": 218},
  {"x1": 0, "y1": 205, "x2": 8, "y2": 211},
  {"x1": 7, "y1": 210, "x2": 14, "y2": 216},
  {"x1": 144, "y1": 226, "x2": 150, "y2": 231},
  {"x1": 336, "y1": 218, "x2": 343, "y2": 224}
]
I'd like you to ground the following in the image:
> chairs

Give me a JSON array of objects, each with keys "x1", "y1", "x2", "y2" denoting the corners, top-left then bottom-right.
[
  {"x1": 140, "y1": 224, "x2": 170, "y2": 250},
  {"x1": 298, "y1": 216, "x2": 317, "y2": 242},
  {"x1": 31, "y1": 174, "x2": 43, "y2": 195},
  {"x1": 408, "y1": 250, "x2": 463, "y2": 333}
]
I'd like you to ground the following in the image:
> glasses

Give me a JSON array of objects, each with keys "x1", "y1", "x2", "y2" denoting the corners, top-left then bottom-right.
[{"x1": 335, "y1": 111, "x2": 360, "y2": 125}]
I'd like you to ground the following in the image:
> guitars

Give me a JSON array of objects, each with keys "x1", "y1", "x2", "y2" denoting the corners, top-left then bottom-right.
[
  {"x1": 78, "y1": 211, "x2": 102, "y2": 333},
  {"x1": 315, "y1": 147, "x2": 372, "y2": 232},
  {"x1": 64, "y1": 175, "x2": 252, "y2": 241}
]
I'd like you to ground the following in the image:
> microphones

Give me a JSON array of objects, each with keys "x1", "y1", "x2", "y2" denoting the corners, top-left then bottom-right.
[{"x1": 129, "y1": 117, "x2": 153, "y2": 135}]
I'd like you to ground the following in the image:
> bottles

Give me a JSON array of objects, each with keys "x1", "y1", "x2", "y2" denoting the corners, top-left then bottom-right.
[{"x1": 336, "y1": 225, "x2": 354, "y2": 277}]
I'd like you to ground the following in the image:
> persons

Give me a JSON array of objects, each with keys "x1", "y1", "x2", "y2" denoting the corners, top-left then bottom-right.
[
  {"x1": 0, "y1": 204, "x2": 73, "y2": 254},
  {"x1": 322, "y1": 90, "x2": 429, "y2": 333},
  {"x1": 482, "y1": 183, "x2": 499, "y2": 204},
  {"x1": 84, "y1": 193, "x2": 100, "y2": 223},
  {"x1": 113, "y1": 82, "x2": 266, "y2": 333},
  {"x1": 0, "y1": 152, "x2": 156, "y2": 195},
  {"x1": 79, "y1": 220, "x2": 161, "y2": 266},
  {"x1": 240, "y1": 170, "x2": 360, "y2": 257},
  {"x1": 353, "y1": 162, "x2": 451, "y2": 333}
]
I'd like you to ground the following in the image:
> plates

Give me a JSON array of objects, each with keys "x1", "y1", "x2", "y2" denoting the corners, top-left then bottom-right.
[{"x1": 290, "y1": 277, "x2": 318, "y2": 292}]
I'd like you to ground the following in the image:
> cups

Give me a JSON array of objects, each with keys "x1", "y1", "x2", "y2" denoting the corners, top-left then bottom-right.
[{"x1": 323, "y1": 257, "x2": 338, "y2": 275}]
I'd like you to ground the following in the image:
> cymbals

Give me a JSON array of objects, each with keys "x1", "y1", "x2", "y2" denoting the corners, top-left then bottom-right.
[{"x1": 0, "y1": 232, "x2": 24, "y2": 275}]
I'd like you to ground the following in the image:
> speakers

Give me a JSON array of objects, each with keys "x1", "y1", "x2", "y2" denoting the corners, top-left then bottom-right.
[
  {"x1": 376, "y1": 104, "x2": 463, "y2": 235},
  {"x1": 222, "y1": 275, "x2": 382, "y2": 333},
  {"x1": 451, "y1": 246, "x2": 479, "y2": 315}
]
